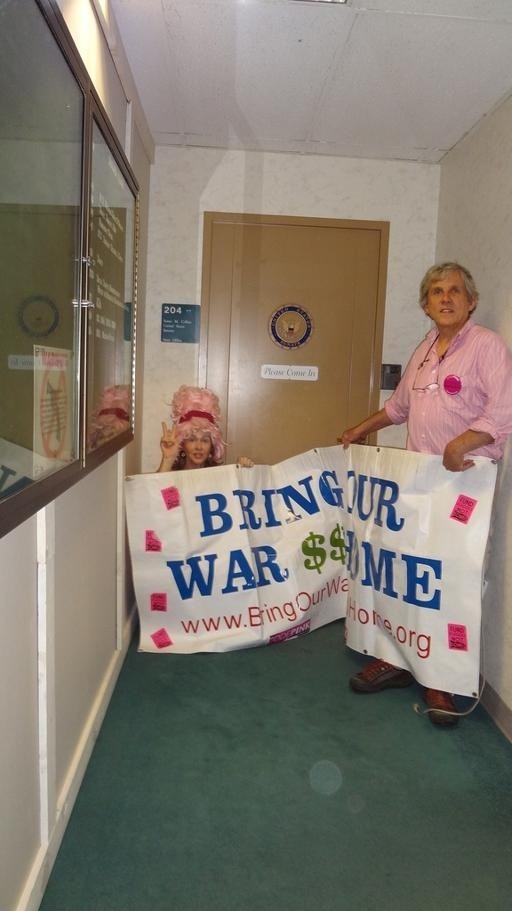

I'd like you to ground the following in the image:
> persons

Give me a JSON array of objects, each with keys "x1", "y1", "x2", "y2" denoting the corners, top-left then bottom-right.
[
  {"x1": 341, "y1": 262, "x2": 512, "y2": 728},
  {"x1": 157, "y1": 386, "x2": 255, "y2": 473},
  {"x1": 87, "y1": 384, "x2": 132, "y2": 449}
]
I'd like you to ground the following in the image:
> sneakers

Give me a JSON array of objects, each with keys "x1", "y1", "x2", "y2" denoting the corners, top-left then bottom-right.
[
  {"x1": 350, "y1": 661, "x2": 414, "y2": 692},
  {"x1": 425, "y1": 689, "x2": 460, "y2": 725}
]
